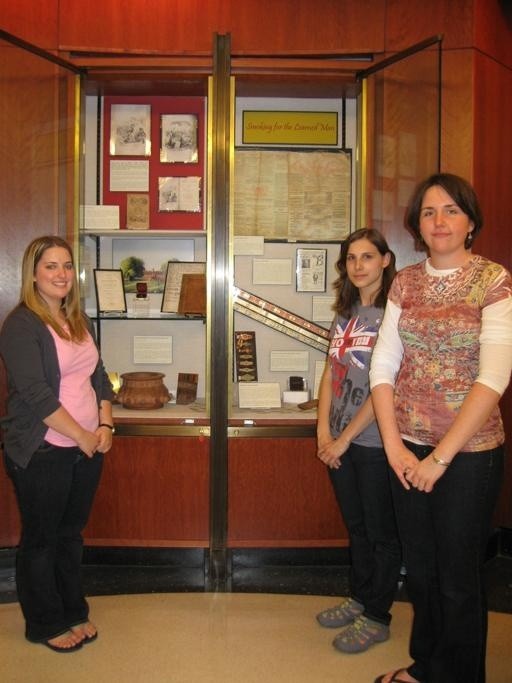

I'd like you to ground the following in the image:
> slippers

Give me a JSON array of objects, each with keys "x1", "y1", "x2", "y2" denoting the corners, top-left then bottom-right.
[
  {"x1": 42, "y1": 634, "x2": 86, "y2": 652},
  {"x1": 72, "y1": 619, "x2": 97, "y2": 643},
  {"x1": 374, "y1": 666, "x2": 417, "y2": 683}
]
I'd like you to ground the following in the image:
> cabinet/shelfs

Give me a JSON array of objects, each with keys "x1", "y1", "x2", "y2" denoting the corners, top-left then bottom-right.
[{"x1": 0, "y1": 0, "x2": 512, "y2": 570}]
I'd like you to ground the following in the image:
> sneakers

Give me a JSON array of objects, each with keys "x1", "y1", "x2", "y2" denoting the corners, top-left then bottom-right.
[
  {"x1": 315, "y1": 596, "x2": 364, "y2": 627},
  {"x1": 331, "y1": 615, "x2": 390, "y2": 653}
]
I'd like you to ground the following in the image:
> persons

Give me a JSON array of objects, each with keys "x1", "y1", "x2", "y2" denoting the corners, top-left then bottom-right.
[
  {"x1": 0, "y1": 234, "x2": 116, "y2": 654},
  {"x1": 313, "y1": 226, "x2": 401, "y2": 657},
  {"x1": 369, "y1": 173, "x2": 512, "y2": 682}
]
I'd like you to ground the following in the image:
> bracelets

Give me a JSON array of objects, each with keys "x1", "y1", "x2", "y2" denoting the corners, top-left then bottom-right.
[
  {"x1": 430, "y1": 450, "x2": 452, "y2": 467},
  {"x1": 98, "y1": 422, "x2": 115, "y2": 434}
]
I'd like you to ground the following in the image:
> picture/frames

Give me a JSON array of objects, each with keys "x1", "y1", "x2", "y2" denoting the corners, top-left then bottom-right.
[
  {"x1": 109, "y1": 103, "x2": 152, "y2": 157},
  {"x1": 93, "y1": 268, "x2": 127, "y2": 313},
  {"x1": 158, "y1": 176, "x2": 202, "y2": 213},
  {"x1": 161, "y1": 261, "x2": 206, "y2": 313},
  {"x1": 159, "y1": 113, "x2": 199, "y2": 164},
  {"x1": 295, "y1": 248, "x2": 327, "y2": 292}
]
